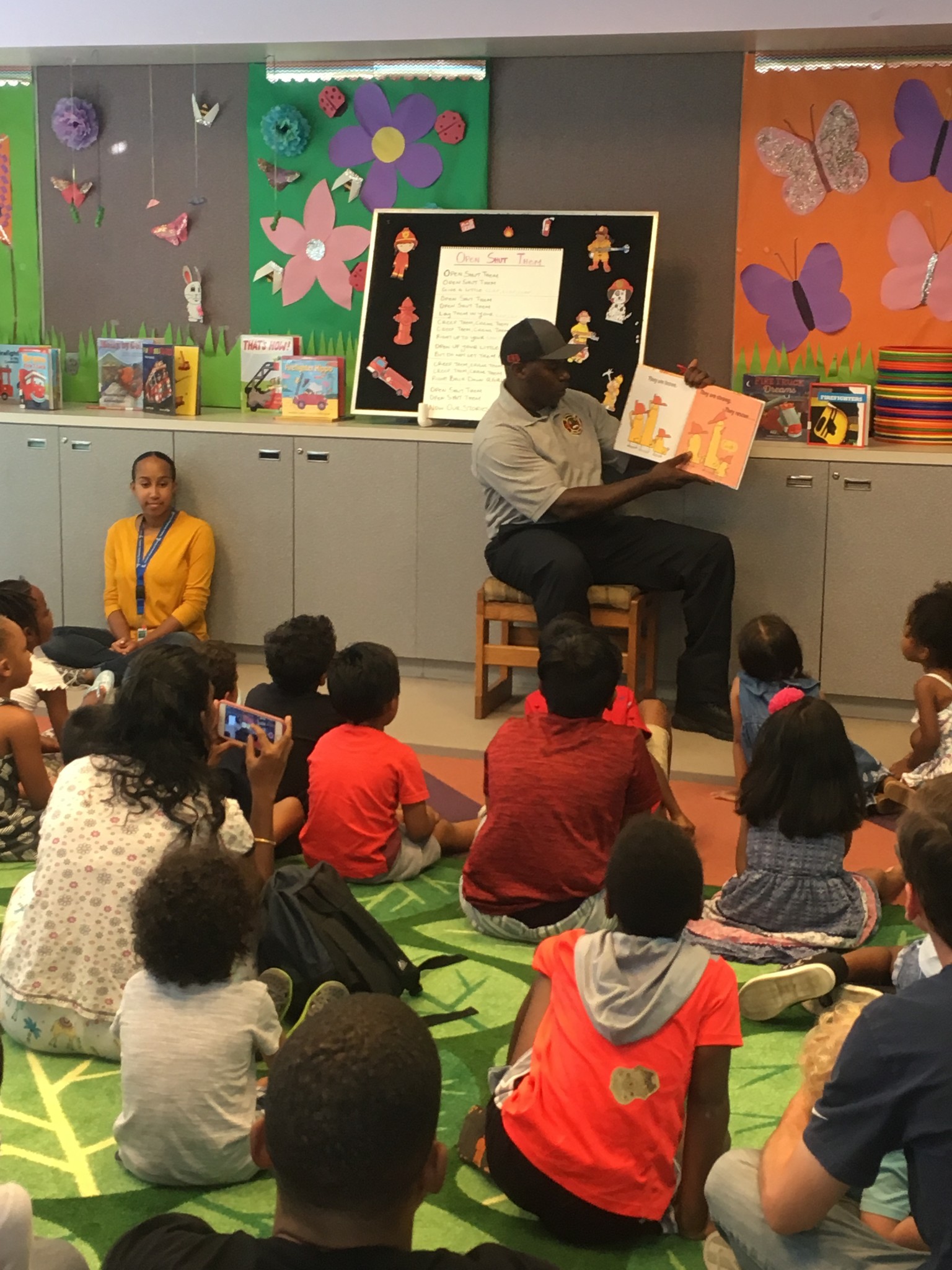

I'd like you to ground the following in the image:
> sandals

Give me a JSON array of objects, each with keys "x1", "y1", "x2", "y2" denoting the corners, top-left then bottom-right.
[
  {"x1": 284, "y1": 980, "x2": 350, "y2": 1042},
  {"x1": 251, "y1": 967, "x2": 292, "y2": 1023}
]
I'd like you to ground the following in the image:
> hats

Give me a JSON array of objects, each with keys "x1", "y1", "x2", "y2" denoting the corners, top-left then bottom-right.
[
  {"x1": 500, "y1": 317, "x2": 590, "y2": 365},
  {"x1": 263, "y1": 992, "x2": 442, "y2": 1198}
]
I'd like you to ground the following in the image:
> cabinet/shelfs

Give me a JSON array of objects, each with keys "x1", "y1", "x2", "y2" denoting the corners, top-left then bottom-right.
[{"x1": 0, "y1": 396, "x2": 952, "y2": 722}]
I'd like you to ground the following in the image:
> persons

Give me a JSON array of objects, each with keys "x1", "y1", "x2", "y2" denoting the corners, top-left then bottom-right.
[
  {"x1": 240, "y1": 612, "x2": 346, "y2": 858},
  {"x1": 713, "y1": 692, "x2": 903, "y2": 962},
  {"x1": 0, "y1": 615, "x2": 55, "y2": 863},
  {"x1": 0, "y1": 644, "x2": 292, "y2": 1065},
  {"x1": 95, "y1": 993, "x2": 561, "y2": 1270},
  {"x1": 453, "y1": 807, "x2": 742, "y2": 1242},
  {"x1": 470, "y1": 317, "x2": 736, "y2": 742},
  {"x1": 459, "y1": 612, "x2": 695, "y2": 944},
  {"x1": 36, "y1": 450, "x2": 214, "y2": 685},
  {"x1": 0, "y1": 576, "x2": 114, "y2": 753},
  {"x1": 109, "y1": 844, "x2": 351, "y2": 1191},
  {"x1": 700, "y1": 774, "x2": 952, "y2": 1270},
  {"x1": 299, "y1": 641, "x2": 484, "y2": 886},
  {"x1": 713, "y1": 614, "x2": 914, "y2": 819},
  {"x1": 200, "y1": 638, "x2": 240, "y2": 706},
  {"x1": 885, "y1": 582, "x2": 952, "y2": 789}
]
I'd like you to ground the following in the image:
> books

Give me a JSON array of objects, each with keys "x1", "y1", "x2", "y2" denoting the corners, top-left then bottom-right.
[
  {"x1": 0, "y1": 345, "x2": 63, "y2": 411},
  {"x1": 97, "y1": 336, "x2": 203, "y2": 416},
  {"x1": 739, "y1": 373, "x2": 823, "y2": 445},
  {"x1": 806, "y1": 380, "x2": 873, "y2": 449},
  {"x1": 239, "y1": 334, "x2": 346, "y2": 424},
  {"x1": 612, "y1": 364, "x2": 767, "y2": 491}
]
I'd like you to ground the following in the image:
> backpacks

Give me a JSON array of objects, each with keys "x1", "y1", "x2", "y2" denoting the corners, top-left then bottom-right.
[{"x1": 259, "y1": 859, "x2": 480, "y2": 1026}]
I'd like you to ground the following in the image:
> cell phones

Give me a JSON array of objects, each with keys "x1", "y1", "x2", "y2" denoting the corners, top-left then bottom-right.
[{"x1": 217, "y1": 698, "x2": 286, "y2": 749}]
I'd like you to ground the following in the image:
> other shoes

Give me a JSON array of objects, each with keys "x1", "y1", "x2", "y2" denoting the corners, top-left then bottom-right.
[
  {"x1": 670, "y1": 695, "x2": 734, "y2": 742},
  {"x1": 457, "y1": 1104, "x2": 491, "y2": 1173},
  {"x1": 83, "y1": 669, "x2": 115, "y2": 705},
  {"x1": 702, "y1": 1229, "x2": 742, "y2": 1270},
  {"x1": 875, "y1": 780, "x2": 917, "y2": 815}
]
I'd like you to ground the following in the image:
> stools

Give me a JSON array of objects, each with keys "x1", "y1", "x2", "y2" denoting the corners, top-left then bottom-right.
[{"x1": 472, "y1": 573, "x2": 658, "y2": 723}]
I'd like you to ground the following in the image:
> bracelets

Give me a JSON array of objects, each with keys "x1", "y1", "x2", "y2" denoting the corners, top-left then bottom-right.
[{"x1": 248, "y1": 837, "x2": 277, "y2": 847}]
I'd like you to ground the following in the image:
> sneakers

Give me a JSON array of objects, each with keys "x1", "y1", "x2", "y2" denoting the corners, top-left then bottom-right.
[
  {"x1": 49, "y1": 662, "x2": 93, "y2": 687},
  {"x1": 801, "y1": 981, "x2": 898, "y2": 1019},
  {"x1": 738, "y1": 952, "x2": 850, "y2": 1022}
]
